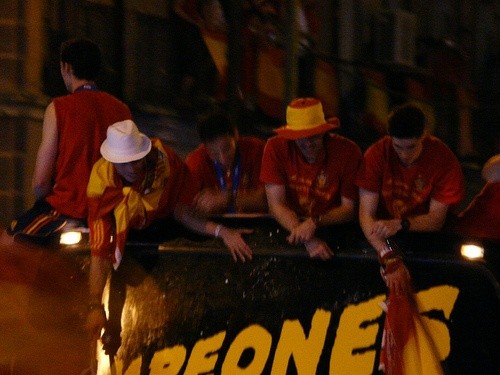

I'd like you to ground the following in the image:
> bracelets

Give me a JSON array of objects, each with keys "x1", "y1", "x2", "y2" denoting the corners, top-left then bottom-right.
[{"x1": 214, "y1": 224, "x2": 223, "y2": 238}]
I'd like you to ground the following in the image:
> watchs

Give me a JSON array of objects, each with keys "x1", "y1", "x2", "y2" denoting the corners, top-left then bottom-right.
[
  {"x1": 400, "y1": 218, "x2": 411, "y2": 231},
  {"x1": 310, "y1": 214, "x2": 323, "y2": 226},
  {"x1": 87, "y1": 303, "x2": 104, "y2": 313}
]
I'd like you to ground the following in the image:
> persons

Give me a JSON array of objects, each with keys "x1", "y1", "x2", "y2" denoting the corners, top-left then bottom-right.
[
  {"x1": 455, "y1": 154, "x2": 500, "y2": 240},
  {"x1": 85, "y1": 119, "x2": 186, "y2": 341},
  {"x1": 259, "y1": 97, "x2": 362, "y2": 261},
  {"x1": 173, "y1": 113, "x2": 266, "y2": 263},
  {"x1": 0, "y1": 38, "x2": 132, "y2": 247},
  {"x1": 353, "y1": 103, "x2": 465, "y2": 296}
]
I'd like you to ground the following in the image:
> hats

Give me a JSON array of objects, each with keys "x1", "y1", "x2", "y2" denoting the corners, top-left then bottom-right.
[
  {"x1": 100, "y1": 120, "x2": 152, "y2": 164},
  {"x1": 272, "y1": 98, "x2": 341, "y2": 139}
]
